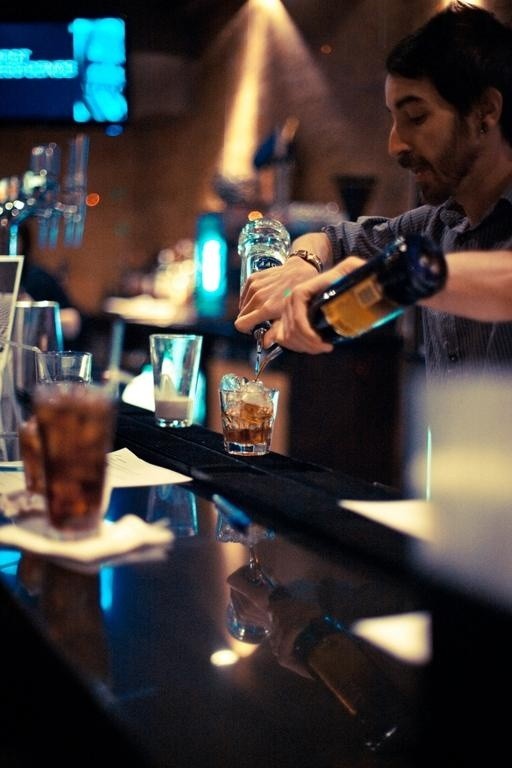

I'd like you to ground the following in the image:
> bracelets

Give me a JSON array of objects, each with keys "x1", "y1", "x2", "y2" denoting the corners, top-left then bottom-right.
[{"x1": 288, "y1": 249, "x2": 324, "y2": 273}]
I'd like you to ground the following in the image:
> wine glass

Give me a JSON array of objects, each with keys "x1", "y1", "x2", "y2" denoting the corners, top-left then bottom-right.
[{"x1": 0, "y1": 301, "x2": 92, "y2": 466}]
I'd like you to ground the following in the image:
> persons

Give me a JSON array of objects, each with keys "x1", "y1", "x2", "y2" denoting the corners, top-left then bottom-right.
[
  {"x1": 0, "y1": 220, "x2": 83, "y2": 352},
  {"x1": 224, "y1": 547, "x2": 458, "y2": 758},
  {"x1": 229, "y1": 2, "x2": 512, "y2": 485}
]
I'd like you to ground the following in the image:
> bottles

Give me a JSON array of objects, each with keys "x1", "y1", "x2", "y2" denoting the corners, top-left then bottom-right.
[
  {"x1": 237, "y1": 216, "x2": 292, "y2": 354},
  {"x1": 278, "y1": 234, "x2": 448, "y2": 355},
  {"x1": 267, "y1": 586, "x2": 415, "y2": 753}
]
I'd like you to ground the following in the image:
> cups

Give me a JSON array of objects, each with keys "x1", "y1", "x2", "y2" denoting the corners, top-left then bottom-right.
[
  {"x1": 19, "y1": 421, "x2": 49, "y2": 504},
  {"x1": 218, "y1": 386, "x2": 281, "y2": 457},
  {"x1": 33, "y1": 379, "x2": 120, "y2": 543},
  {"x1": 148, "y1": 333, "x2": 204, "y2": 431},
  {"x1": 146, "y1": 484, "x2": 200, "y2": 539}
]
